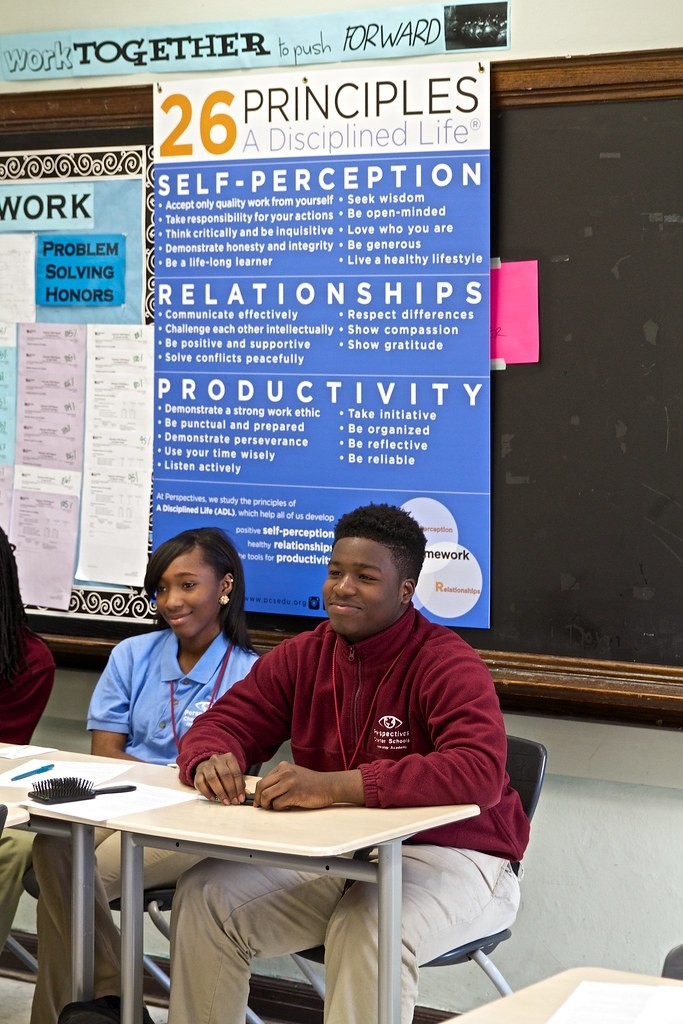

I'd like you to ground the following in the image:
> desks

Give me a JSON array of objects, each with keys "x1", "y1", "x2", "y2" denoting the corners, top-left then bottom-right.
[{"x1": 0, "y1": 742, "x2": 481, "y2": 1024}]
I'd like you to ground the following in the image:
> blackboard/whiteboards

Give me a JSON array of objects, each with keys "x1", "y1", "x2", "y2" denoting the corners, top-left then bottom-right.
[{"x1": 0, "y1": 41, "x2": 683, "y2": 714}]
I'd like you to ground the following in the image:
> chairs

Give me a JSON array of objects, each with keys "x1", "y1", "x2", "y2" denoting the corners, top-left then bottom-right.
[
  {"x1": 289, "y1": 735, "x2": 547, "y2": 1005},
  {"x1": 22, "y1": 762, "x2": 267, "y2": 1024}
]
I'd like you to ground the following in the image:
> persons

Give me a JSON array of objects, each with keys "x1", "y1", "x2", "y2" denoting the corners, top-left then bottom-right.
[
  {"x1": 30, "y1": 527, "x2": 261, "y2": 1024},
  {"x1": 0, "y1": 518, "x2": 55, "y2": 959},
  {"x1": 176, "y1": 501, "x2": 522, "y2": 1023}
]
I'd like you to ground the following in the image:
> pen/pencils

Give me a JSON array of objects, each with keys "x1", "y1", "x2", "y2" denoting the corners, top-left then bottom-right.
[{"x1": 11, "y1": 764, "x2": 55, "y2": 781}]
